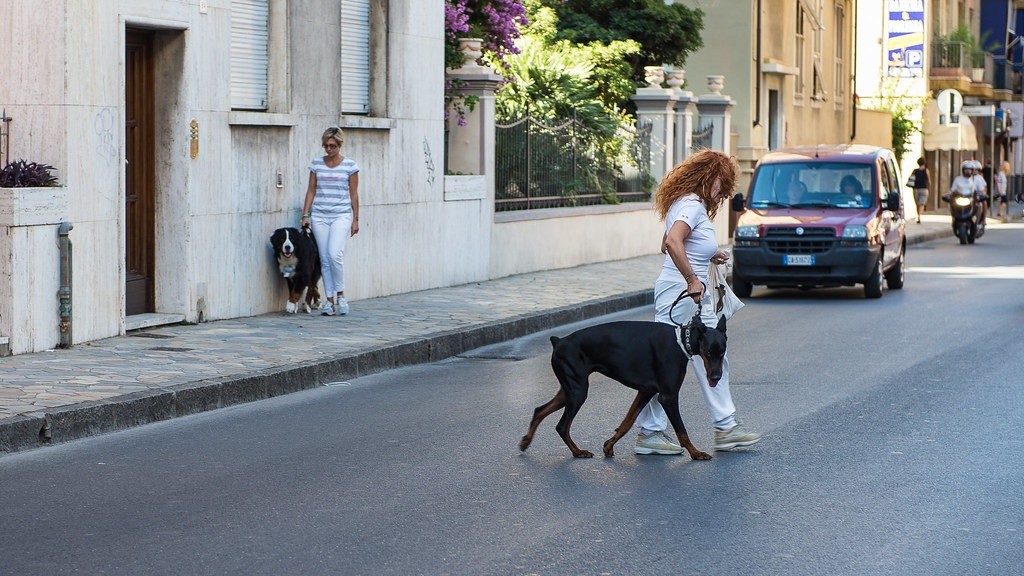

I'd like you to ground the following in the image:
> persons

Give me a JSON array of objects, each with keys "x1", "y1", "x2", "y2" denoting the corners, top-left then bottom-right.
[
  {"x1": 911, "y1": 157, "x2": 931, "y2": 223},
  {"x1": 632, "y1": 150, "x2": 761, "y2": 454},
  {"x1": 300, "y1": 126, "x2": 360, "y2": 316},
  {"x1": 786, "y1": 182, "x2": 805, "y2": 205},
  {"x1": 981, "y1": 160, "x2": 1010, "y2": 217},
  {"x1": 945, "y1": 159, "x2": 988, "y2": 225},
  {"x1": 840, "y1": 175, "x2": 863, "y2": 205}
]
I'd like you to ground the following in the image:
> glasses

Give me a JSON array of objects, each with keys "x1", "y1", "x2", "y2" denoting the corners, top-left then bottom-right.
[
  {"x1": 322, "y1": 143, "x2": 337, "y2": 149},
  {"x1": 844, "y1": 183, "x2": 852, "y2": 187}
]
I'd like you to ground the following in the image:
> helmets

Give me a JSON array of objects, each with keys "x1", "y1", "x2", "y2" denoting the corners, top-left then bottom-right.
[
  {"x1": 962, "y1": 160, "x2": 974, "y2": 169},
  {"x1": 973, "y1": 160, "x2": 983, "y2": 170}
]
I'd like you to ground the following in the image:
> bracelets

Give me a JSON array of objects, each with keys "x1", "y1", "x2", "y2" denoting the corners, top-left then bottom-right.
[
  {"x1": 685, "y1": 273, "x2": 695, "y2": 280},
  {"x1": 302, "y1": 213, "x2": 310, "y2": 217}
]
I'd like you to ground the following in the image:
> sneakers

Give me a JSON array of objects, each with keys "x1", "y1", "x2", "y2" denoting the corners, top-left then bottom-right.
[
  {"x1": 713, "y1": 425, "x2": 761, "y2": 451},
  {"x1": 321, "y1": 303, "x2": 337, "y2": 316},
  {"x1": 337, "y1": 297, "x2": 350, "y2": 314},
  {"x1": 635, "y1": 431, "x2": 684, "y2": 455}
]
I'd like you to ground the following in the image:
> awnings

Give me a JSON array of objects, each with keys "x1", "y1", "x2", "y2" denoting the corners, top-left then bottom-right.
[{"x1": 922, "y1": 99, "x2": 979, "y2": 151}]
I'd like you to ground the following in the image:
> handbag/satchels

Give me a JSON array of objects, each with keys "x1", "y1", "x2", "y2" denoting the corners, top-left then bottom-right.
[
  {"x1": 709, "y1": 249, "x2": 745, "y2": 320},
  {"x1": 906, "y1": 173, "x2": 917, "y2": 186}
]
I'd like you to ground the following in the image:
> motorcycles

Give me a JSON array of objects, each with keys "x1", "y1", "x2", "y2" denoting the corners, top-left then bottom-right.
[{"x1": 941, "y1": 193, "x2": 989, "y2": 244}]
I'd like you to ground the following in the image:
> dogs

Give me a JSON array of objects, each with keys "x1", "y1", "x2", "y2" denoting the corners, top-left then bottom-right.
[
  {"x1": 269, "y1": 227, "x2": 322, "y2": 314},
  {"x1": 519, "y1": 314, "x2": 729, "y2": 460}
]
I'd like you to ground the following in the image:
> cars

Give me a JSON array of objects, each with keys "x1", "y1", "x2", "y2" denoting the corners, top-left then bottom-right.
[{"x1": 731, "y1": 141, "x2": 907, "y2": 301}]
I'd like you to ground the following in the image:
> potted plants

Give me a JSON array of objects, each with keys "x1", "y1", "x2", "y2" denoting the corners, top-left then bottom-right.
[
  {"x1": 929, "y1": 24, "x2": 972, "y2": 75},
  {"x1": 966, "y1": 28, "x2": 1000, "y2": 81},
  {"x1": 0, "y1": 159, "x2": 69, "y2": 226}
]
